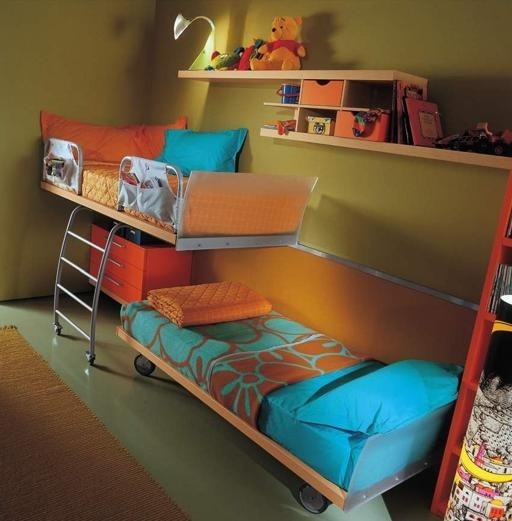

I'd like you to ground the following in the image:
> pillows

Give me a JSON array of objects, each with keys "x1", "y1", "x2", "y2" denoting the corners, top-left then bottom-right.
[
  {"x1": 295, "y1": 341, "x2": 461, "y2": 438},
  {"x1": 37, "y1": 114, "x2": 250, "y2": 174}
]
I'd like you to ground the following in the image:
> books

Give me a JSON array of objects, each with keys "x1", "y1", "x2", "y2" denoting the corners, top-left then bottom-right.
[
  {"x1": 401, "y1": 95, "x2": 426, "y2": 145},
  {"x1": 404, "y1": 98, "x2": 444, "y2": 147},
  {"x1": 486, "y1": 264, "x2": 512, "y2": 315},
  {"x1": 396, "y1": 81, "x2": 424, "y2": 143}
]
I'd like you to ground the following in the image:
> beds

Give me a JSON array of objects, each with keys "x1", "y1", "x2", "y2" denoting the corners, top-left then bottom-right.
[
  {"x1": 31, "y1": 156, "x2": 323, "y2": 255},
  {"x1": 112, "y1": 277, "x2": 463, "y2": 515}
]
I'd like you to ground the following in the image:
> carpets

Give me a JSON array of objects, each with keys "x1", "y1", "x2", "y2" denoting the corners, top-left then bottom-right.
[{"x1": 1, "y1": 324, "x2": 190, "y2": 521}]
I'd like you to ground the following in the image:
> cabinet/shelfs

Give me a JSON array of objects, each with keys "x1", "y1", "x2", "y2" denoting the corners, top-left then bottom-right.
[{"x1": 81, "y1": 222, "x2": 192, "y2": 309}]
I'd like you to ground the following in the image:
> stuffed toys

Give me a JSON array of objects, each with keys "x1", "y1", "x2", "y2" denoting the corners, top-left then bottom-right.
[
  {"x1": 203, "y1": 37, "x2": 268, "y2": 71},
  {"x1": 250, "y1": 15, "x2": 308, "y2": 69}
]
